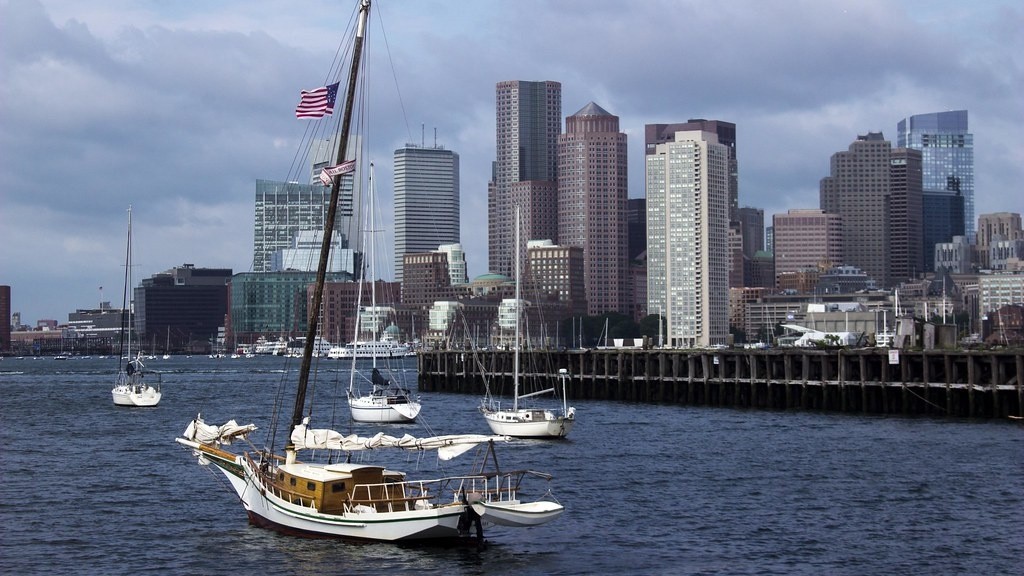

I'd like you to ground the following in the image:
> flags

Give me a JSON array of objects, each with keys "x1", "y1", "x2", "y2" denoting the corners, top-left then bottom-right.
[{"x1": 296, "y1": 81, "x2": 339, "y2": 120}]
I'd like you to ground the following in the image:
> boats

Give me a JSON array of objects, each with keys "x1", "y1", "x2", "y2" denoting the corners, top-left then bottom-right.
[{"x1": 236, "y1": 330, "x2": 408, "y2": 359}]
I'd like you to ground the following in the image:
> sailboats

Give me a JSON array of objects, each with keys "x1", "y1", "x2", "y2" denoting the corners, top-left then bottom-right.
[
  {"x1": 344, "y1": 160, "x2": 423, "y2": 424},
  {"x1": 479, "y1": 203, "x2": 577, "y2": 440},
  {"x1": 174, "y1": 0, "x2": 568, "y2": 546},
  {"x1": 112, "y1": 204, "x2": 164, "y2": 407}
]
